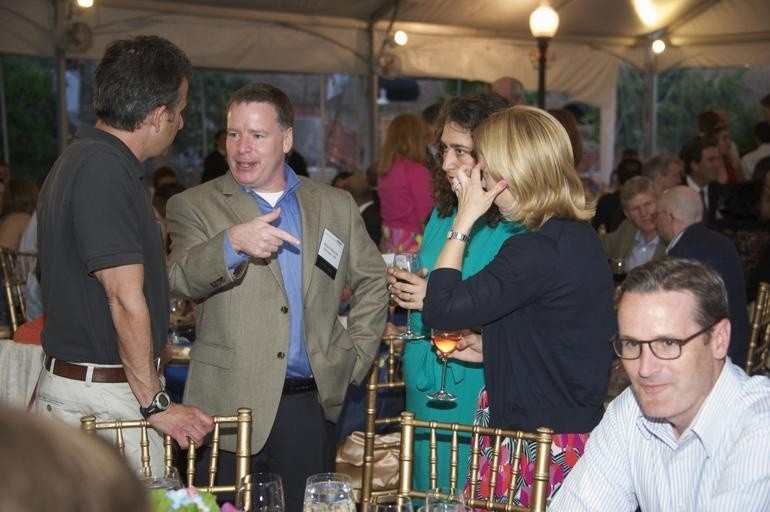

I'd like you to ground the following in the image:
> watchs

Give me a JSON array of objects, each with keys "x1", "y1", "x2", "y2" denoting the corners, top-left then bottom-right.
[
  {"x1": 442, "y1": 228, "x2": 474, "y2": 245},
  {"x1": 137, "y1": 391, "x2": 172, "y2": 419}
]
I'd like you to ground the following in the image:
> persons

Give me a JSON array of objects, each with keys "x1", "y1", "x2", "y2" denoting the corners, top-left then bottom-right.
[
  {"x1": 546, "y1": 258, "x2": 770, "y2": 510},
  {"x1": 421, "y1": 102, "x2": 623, "y2": 511},
  {"x1": 29, "y1": 35, "x2": 218, "y2": 490},
  {"x1": 0, "y1": 76, "x2": 769, "y2": 443},
  {"x1": 385, "y1": 90, "x2": 527, "y2": 510}
]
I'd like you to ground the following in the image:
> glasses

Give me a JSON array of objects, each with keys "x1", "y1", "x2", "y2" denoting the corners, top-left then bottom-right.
[{"x1": 609, "y1": 322, "x2": 712, "y2": 359}]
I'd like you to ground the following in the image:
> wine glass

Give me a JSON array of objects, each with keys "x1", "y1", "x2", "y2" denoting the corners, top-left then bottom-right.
[
  {"x1": 428, "y1": 320, "x2": 459, "y2": 410},
  {"x1": 389, "y1": 246, "x2": 425, "y2": 348},
  {"x1": 612, "y1": 254, "x2": 628, "y2": 284},
  {"x1": 167, "y1": 294, "x2": 186, "y2": 345}
]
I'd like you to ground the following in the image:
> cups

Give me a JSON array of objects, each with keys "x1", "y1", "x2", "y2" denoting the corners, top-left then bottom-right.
[
  {"x1": 371, "y1": 489, "x2": 412, "y2": 512},
  {"x1": 241, "y1": 471, "x2": 289, "y2": 511},
  {"x1": 426, "y1": 483, "x2": 467, "y2": 511},
  {"x1": 302, "y1": 471, "x2": 355, "y2": 512}
]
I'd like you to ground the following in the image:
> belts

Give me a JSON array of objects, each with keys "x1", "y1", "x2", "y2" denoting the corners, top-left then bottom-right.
[
  {"x1": 282, "y1": 378, "x2": 317, "y2": 394},
  {"x1": 43, "y1": 357, "x2": 127, "y2": 382}
]
